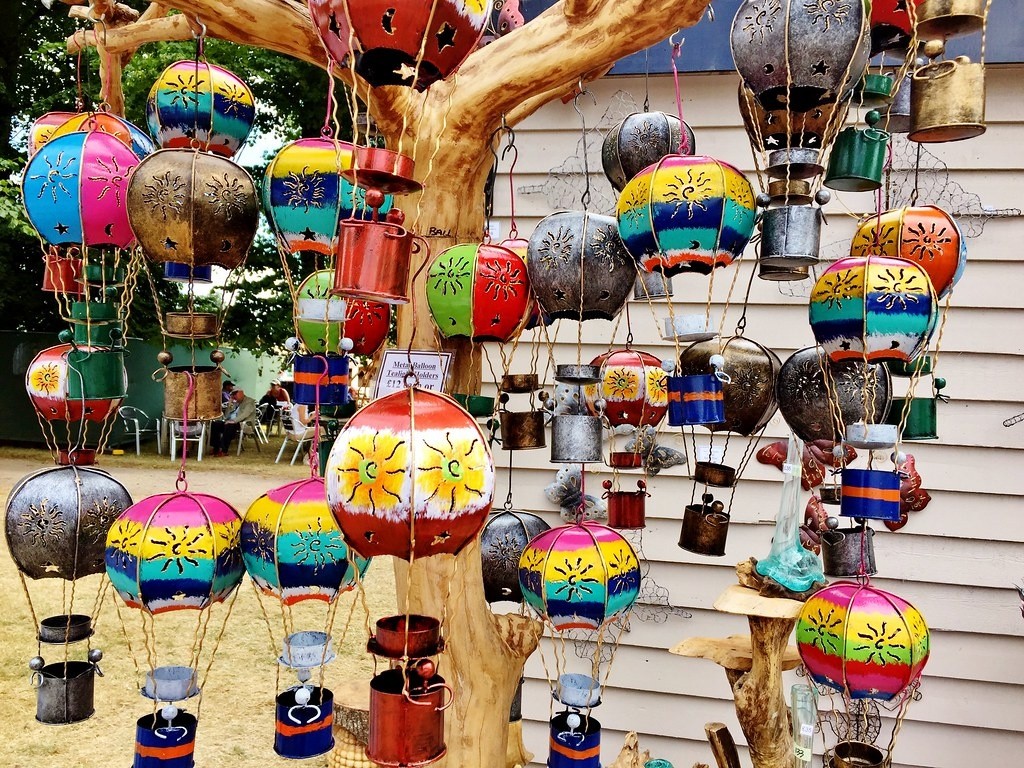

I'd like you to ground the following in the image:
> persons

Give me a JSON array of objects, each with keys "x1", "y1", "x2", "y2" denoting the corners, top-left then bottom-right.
[{"x1": 209, "y1": 377, "x2": 356, "y2": 466}]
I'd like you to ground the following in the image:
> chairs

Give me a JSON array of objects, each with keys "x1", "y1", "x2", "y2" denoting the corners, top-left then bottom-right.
[{"x1": 119, "y1": 403, "x2": 314, "y2": 467}]
